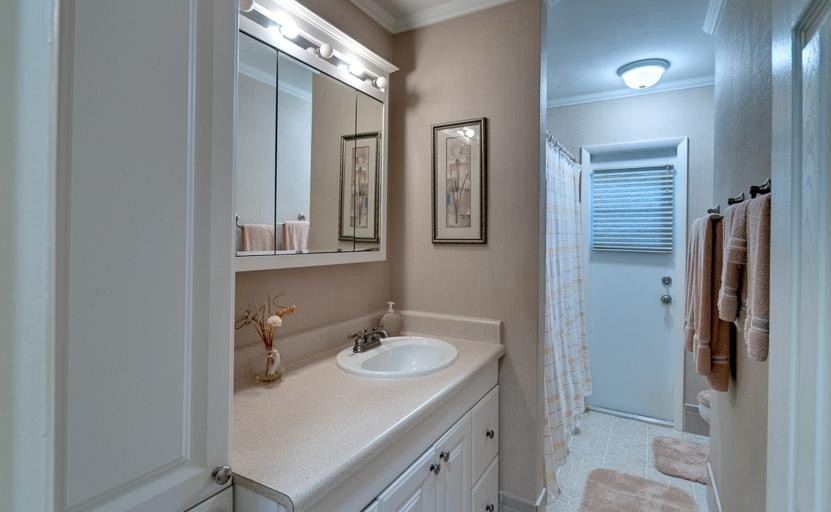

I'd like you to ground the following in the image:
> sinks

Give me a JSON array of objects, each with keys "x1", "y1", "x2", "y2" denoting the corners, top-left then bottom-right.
[{"x1": 334, "y1": 336, "x2": 459, "y2": 378}]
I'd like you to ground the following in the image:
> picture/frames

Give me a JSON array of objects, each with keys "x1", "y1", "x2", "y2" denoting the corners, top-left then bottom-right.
[{"x1": 430, "y1": 117, "x2": 493, "y2": 250}]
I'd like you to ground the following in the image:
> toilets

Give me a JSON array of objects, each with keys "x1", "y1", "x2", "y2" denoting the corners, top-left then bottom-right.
[{"x1": 696, "y1": 390, "x2": 711, "y2": 423}]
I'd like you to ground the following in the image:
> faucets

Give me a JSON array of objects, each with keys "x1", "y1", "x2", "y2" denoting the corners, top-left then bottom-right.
[{"x1": 363, "y1": 327, "x2": 387, "y2": 343}]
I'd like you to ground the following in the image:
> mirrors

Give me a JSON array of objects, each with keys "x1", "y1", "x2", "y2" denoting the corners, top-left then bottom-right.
[{"x1": 233, "y1": 30, "x2": 387, "y2": 260}]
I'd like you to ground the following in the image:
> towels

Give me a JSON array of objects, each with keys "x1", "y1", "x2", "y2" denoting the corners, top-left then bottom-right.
[{"x1": 676, "y1": 191, "x2": 774, "y2": 393}]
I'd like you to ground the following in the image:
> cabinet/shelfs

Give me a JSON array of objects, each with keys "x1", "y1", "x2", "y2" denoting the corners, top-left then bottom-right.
[
  {"x1": 472, "y1": 384, "x2": 502, "y2": 512},
  {"x1": 376, "y1": 413, "x2": 473, "y2": 512}
]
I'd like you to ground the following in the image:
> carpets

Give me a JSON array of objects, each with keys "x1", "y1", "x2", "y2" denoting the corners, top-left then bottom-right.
[{"x1": 576, "y1": 430, "x2": 717, "y2": 512}]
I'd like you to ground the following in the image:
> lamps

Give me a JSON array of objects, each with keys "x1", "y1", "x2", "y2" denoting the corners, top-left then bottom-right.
[{"x1": 617, "y1": 55, "x2": 673, "y2": 92}]
